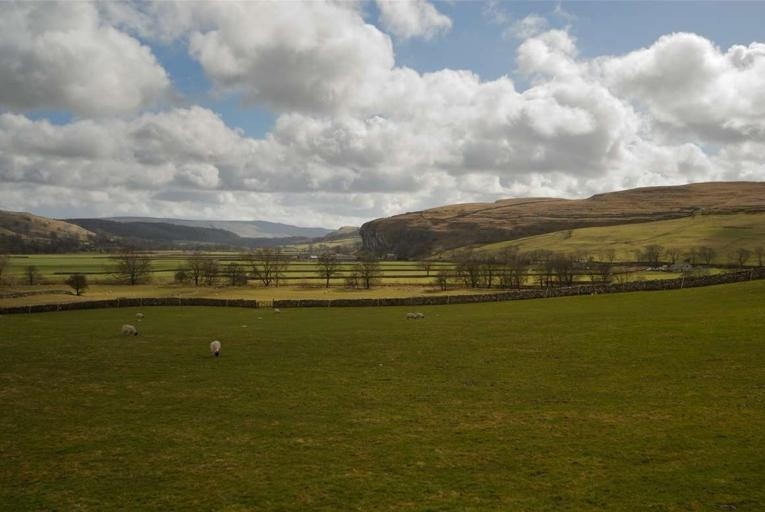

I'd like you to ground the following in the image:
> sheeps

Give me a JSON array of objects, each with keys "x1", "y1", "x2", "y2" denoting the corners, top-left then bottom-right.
[
  {"x1": 136, "y1": 312, "x2": 144, "y2": 318},
  {"x1": 416, "y1": 313, "x2": 424, "y2": 318},
  {"x1": 407, "y1": 312, "x2": 417, "y2": 319},
  {"x1": 121, "y1": 324, "x2": 138, "y2": 337},
  {"x1": 210, "y1": 340, "x2": 220, "y2": 357}
]
